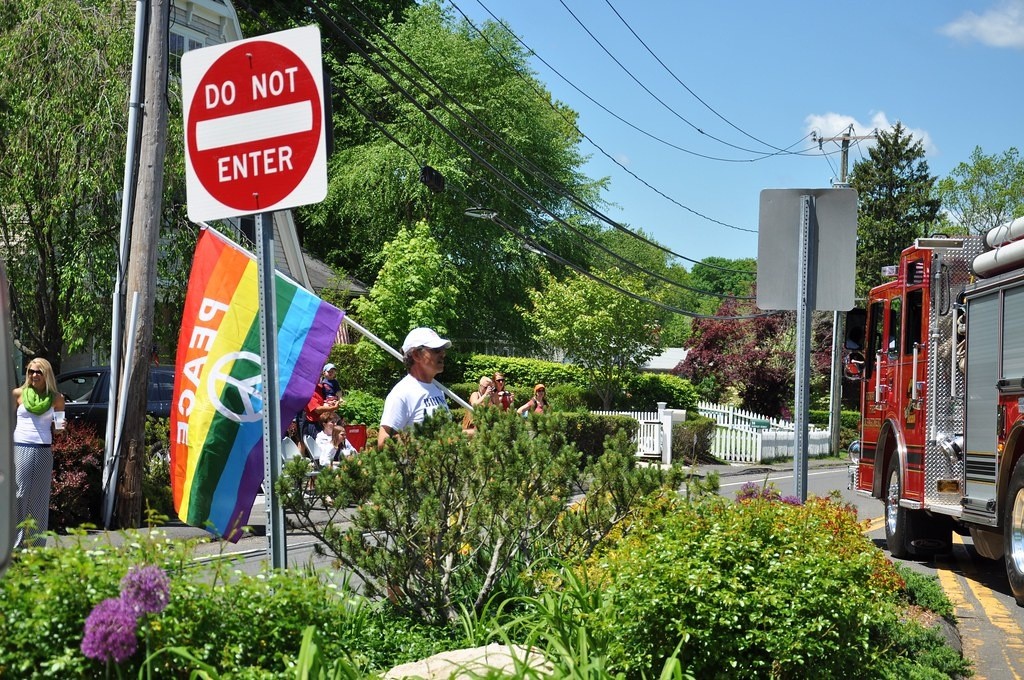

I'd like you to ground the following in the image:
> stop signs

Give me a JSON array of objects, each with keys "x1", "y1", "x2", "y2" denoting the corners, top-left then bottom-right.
[{"x1": 180, "y1": 24, "x2": 328, "y2": 224}]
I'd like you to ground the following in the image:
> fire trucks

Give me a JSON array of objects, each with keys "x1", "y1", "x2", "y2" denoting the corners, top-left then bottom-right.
[{"x1": 837, "y1": 216, "x2": 1024, "y2": 603}]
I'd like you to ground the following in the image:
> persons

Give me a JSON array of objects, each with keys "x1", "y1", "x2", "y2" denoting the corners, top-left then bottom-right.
[
  {"x1": 12, "y1": 358, "x2": 68, "y2": 548},
  {"x1": 295, "y1": 363, "x2": 357, "y2": 506},
  {"x1": 462, "y1": 376, "x2": 497, "y2": 432},
  {"x1": 378, "y1": 327, "x2": 475, "y2": 449},
  {"x1": 517, "y1": 384, "x2": 551, "y2": 417},
  {"x1": 492, "y1": 371, "x2": 514, "y2": 411}
]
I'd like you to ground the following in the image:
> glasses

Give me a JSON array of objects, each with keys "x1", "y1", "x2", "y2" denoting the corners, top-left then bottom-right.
[
  {"x1": 495, "y1": 379, "x2": 505, "y2": 382},
  {"x1": 418, "y1": 347, "x2": 442, "y2": 354},
  {"x1": 29, "y1": 369, "x2": 42, "y2": 375},
  {"x1": 537, "y1": 390, "x2": 545, "y2": 392}
]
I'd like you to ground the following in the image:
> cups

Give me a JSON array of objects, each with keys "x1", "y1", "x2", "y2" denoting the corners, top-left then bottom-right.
[{"x1": 53, "y1": 411, "x2": 65, "y2": 429}]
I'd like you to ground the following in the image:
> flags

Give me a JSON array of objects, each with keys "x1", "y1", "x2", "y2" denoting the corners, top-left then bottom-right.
[{"x1": 170, "y1": 229, "x2": 343, "y2": 544}]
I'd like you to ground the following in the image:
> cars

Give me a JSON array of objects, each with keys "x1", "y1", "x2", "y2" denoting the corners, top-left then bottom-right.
[{"x1": 56, "y1": 367, "x2": 177, "y2": 425}]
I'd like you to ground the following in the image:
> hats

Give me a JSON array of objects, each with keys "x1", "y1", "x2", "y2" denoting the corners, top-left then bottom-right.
[
  {"x1": 534, "y1": 384, "x2": 545, "y2": 390},
  {"x1": 401, "y1": 327, "x2": 452, "y2": 353},
  {"x1": 323, "y1": 363, "x2": 337, "y2": 372}
]
